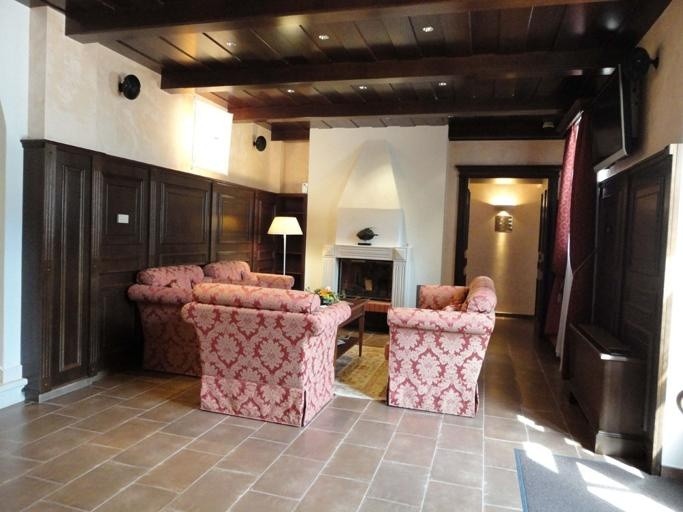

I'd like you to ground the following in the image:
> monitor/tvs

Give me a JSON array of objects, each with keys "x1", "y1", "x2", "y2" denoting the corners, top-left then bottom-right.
[{"x1": 590, "y1": 63, "x2": 634, "y2": 174}]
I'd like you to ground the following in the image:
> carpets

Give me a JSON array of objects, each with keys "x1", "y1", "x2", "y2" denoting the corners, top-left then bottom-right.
[{"x1": 514, "y1": 448, "x2": 683, "y2": 511}]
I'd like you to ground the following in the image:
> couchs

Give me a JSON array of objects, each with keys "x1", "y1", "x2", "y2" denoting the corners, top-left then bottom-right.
[{"x1": 386, "y1": 276, "x2": 497, "y2": 418}]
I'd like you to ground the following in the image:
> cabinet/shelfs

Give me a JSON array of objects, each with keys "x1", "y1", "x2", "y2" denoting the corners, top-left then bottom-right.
[
  {"x1": 147, "y1": 166, "x2": 255, "y2": 273},
  {"x1": 560, "y1": 141, "x2": 682, "y2": 476},
  {"x1": 254, "y1": 190, "x2": 306, "y2": 273},
  {"x1": 19, "y1": 139, "x2": 150, "y2": 395}
]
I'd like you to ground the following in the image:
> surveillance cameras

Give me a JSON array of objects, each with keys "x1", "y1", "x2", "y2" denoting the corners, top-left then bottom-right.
[{"x1": 542, "y1": 121, "x2": 555, "y2": 132}]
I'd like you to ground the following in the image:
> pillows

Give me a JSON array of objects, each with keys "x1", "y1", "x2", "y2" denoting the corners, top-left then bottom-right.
[{"x1": 442, "y1": 302, "x2": 462, "y2": 312}]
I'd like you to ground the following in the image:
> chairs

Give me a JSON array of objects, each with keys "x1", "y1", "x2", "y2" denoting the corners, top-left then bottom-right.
[{"x1": 128, "y1": 259, "x2": 353, "y2": 427}]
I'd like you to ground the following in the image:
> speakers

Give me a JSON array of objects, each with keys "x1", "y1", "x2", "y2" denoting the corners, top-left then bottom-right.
[
  {"x1": 623, "y1": 46, "x2": 650, "y2": 80},
  {"x1": 256, "y1": 136, "x2": 266, "y2": 151},
  {"x1": 122, "y1": 74, "x2": 141, "y2": 100}
]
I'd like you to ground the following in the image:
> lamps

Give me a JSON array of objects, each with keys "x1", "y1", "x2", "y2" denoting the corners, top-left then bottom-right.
[
  {"x1": 494, "y1": 205, "x2": 512, "y2": 233},
  {"x1": 267, "y1": 217, "x2": 304, "y2": 275}
]
